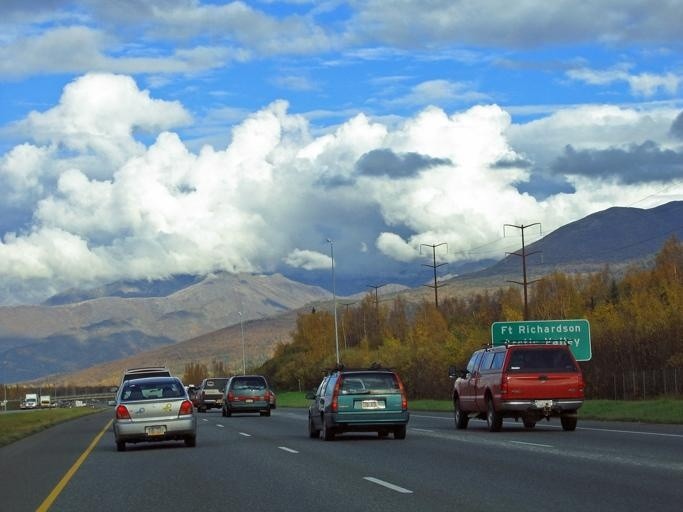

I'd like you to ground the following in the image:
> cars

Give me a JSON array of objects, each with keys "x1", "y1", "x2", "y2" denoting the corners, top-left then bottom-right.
[
  {"x1": 113, "y1": 376, "x2": 197, "y2": 450},
  {"x1": 50, "y1": 402, "x2": 71, "y2": 408},
  {"x1": 19, "y1": 400, "x2": 26, "y2": 409},
  {"x1": 83, "y1": 400, "x2": 93, "y2": 407}
]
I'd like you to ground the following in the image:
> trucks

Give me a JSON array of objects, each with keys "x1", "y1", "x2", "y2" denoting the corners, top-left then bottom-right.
[
  {"x1": 71, "y1": 400, "x2": 83, "y2": 407},
  {"x1": 26, "y1": 393, "x2": 51, "y2": 408}
]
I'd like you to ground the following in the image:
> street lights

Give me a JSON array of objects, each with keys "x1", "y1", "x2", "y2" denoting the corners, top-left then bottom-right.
[
  {"x1": 237, "y1": 310, "x2": 246, "y2": 375},
  {"x1": 326, "y1": 238, "x2": 340, "y2": 367}
]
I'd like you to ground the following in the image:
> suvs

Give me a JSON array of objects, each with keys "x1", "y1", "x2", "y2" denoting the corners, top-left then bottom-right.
[
  {"x1": 449, "y1": 340, "x2": 584, "y2": 431},
  {"x1": 187, "y1": 375, "x2": 276, "y2": 417},
  {"x1": 307, "y1": 364, "x2": 410, "y2": 440}
]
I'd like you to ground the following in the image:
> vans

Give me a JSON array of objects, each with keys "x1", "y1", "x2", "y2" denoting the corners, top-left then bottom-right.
[{"x1": 116, "y1": 367, "x2": 178, "y2": 399}]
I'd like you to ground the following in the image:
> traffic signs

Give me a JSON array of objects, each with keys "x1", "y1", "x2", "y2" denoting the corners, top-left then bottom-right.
[{"x1": 491, "y1": 319, "x2": 592, "y2": 362}]
